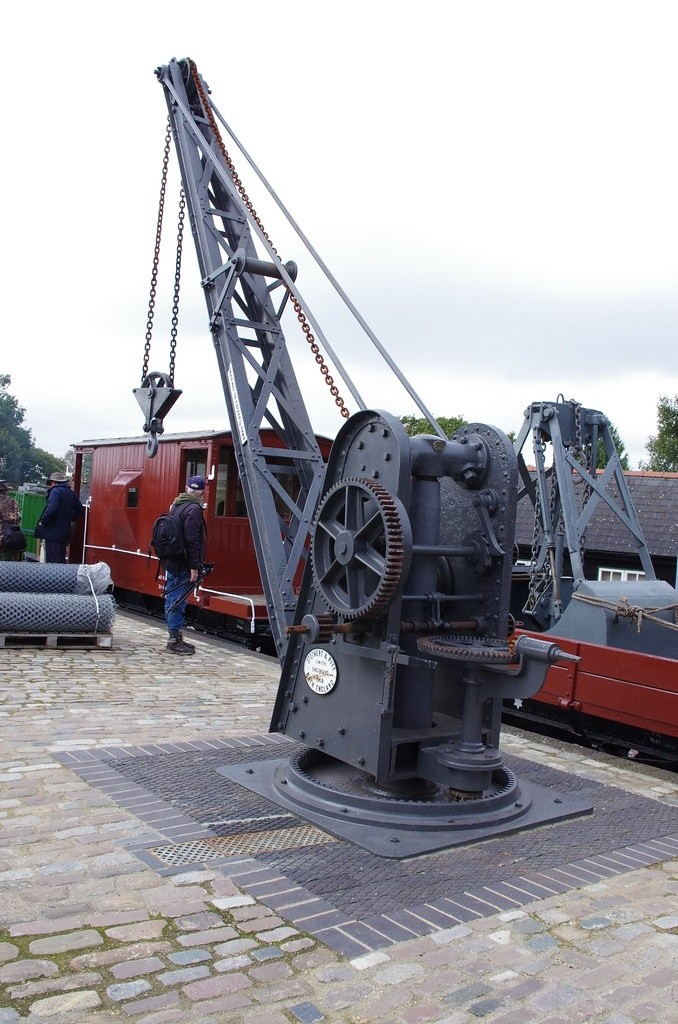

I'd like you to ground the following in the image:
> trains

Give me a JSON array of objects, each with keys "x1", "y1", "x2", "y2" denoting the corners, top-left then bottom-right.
[{"x1": 8, "y1": 392, "x2": 678, "y2": 737}]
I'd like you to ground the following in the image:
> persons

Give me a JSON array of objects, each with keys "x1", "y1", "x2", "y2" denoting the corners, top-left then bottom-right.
[
  {"x1": 0, "y1": 482, "x2": 22, "y2": 561},
  {"x1": 33, "y1": 472, "x2": 83, "y2": 563},
  {"x1": 165, "y1": 476, "x2": 208, "y2": 656}
]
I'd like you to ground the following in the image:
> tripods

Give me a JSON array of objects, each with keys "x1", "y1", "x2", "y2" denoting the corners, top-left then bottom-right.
[{"x1": 160, "y1": 570, "x2": 206, "y2": 614}]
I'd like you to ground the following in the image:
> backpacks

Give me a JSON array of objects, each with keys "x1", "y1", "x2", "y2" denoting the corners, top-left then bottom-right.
[
  {"x1": 2, "y1": 526, "x2": 28, "y2": 554},
  {"x1": 150, "y1": 501, "x2": 194, "y2": 573}
]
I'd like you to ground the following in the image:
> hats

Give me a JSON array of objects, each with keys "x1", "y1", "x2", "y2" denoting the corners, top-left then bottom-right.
[
  {"x1": 188, "y1": 476, "x2": 205, "y2": 489},
  {"x1": 46, "y1": 473, "x2": 70, "y2": 486},
  {"x1": 0, "y1": 480, "x2": 13, "y2": 490}
]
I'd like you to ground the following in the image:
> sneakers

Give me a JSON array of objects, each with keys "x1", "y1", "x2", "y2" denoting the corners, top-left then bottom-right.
[{"x1": 167, "y1": 636, "x2": 196, "y2": 655}]
